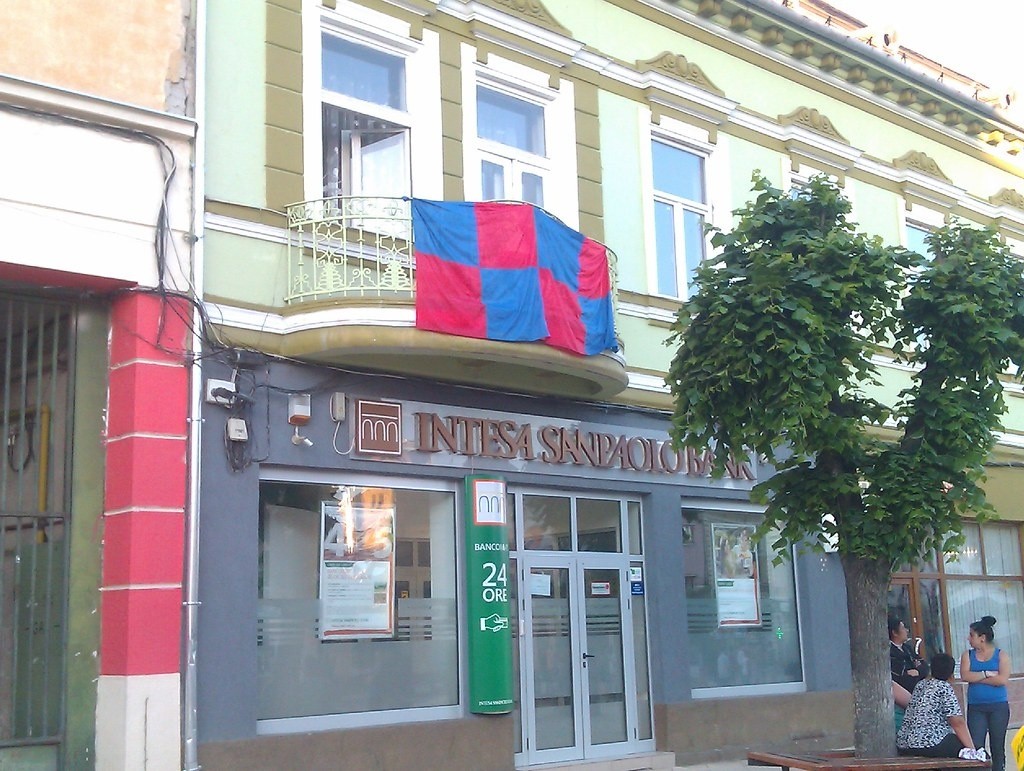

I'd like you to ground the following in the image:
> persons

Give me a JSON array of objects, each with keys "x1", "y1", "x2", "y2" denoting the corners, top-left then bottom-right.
[
  {"x1": 960, "y1": 615, "x2": 1010, "y2": 771},
  {"x1": 888, "y1": 615, "x2": 975, "y2": 758}
]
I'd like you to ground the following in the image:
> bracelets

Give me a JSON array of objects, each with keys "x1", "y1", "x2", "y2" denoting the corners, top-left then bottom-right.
[{"x1": 982, "y1": 670, "x2": 988, "y2": 678}]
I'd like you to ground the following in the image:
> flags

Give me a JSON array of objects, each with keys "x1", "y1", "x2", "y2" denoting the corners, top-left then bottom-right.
[{"x1": 406, "y1": 197, "x2": 618, "y2": 356}]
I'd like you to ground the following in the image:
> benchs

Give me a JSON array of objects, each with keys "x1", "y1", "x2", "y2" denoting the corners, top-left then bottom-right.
[{"x1": 748, "y1": 747, "x2": 994, "y2": 771}]
